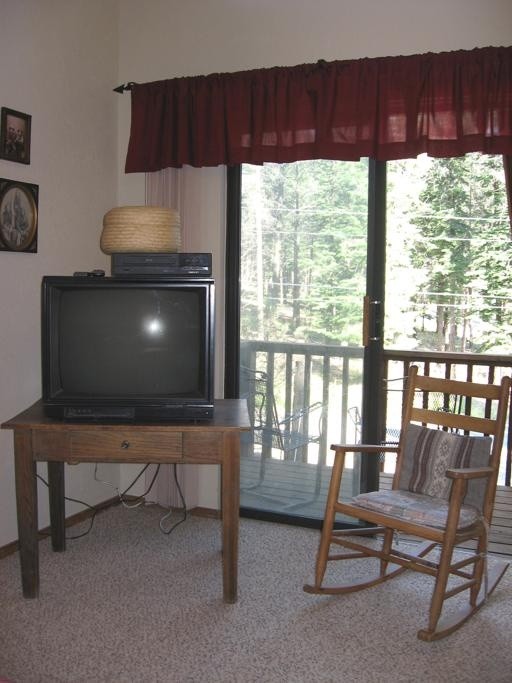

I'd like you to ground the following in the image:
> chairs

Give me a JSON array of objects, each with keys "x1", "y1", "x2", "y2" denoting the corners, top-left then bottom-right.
[
  {"x1": 341, "y1": 378, "x2": 465, "y2": 501},
  {"x1": 239, "y1": 364, "x2": 327, "y2": 513},
  {"x1": 300, "y1": 365, "x2": 510, "y2": 641}
]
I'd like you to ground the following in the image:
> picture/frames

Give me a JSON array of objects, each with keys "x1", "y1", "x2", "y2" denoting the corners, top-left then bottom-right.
[
  {"x1": 0, "y1": 177, "x2": 40, "y2": 253},
  {"x1": 0, "y1": 106, "x2": 32, "y2": 166}
]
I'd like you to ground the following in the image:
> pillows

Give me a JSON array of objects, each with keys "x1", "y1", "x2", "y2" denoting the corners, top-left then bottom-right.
[{"x1": 398, "y1": 421, "x2": 492, "y2": 512}]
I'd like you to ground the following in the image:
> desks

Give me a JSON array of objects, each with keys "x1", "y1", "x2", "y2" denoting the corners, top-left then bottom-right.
[{"x1": 2, "y1": 398, "x2": 252, "y2": 604}]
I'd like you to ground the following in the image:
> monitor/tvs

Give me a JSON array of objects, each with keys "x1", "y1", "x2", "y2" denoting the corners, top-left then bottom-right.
[{"x1": 41, "y1": 276, "x2": 215, "y2": 421}]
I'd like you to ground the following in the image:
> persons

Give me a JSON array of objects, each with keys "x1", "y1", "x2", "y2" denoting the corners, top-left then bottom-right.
[
  {"x1": 2, "y1": 190, "x2": 30, "y2": 247},
  {"x1": 7, "y1": 125, "x2": 24, "y2": 161}
]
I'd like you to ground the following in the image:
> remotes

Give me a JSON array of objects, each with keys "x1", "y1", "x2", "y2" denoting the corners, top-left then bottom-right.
[
  {"x1": 92, "y1": 270, "x2": 105, "y2": 276},
  {"x1": 74, "y1": 272, "x2": 92, "y2": 276}
]
